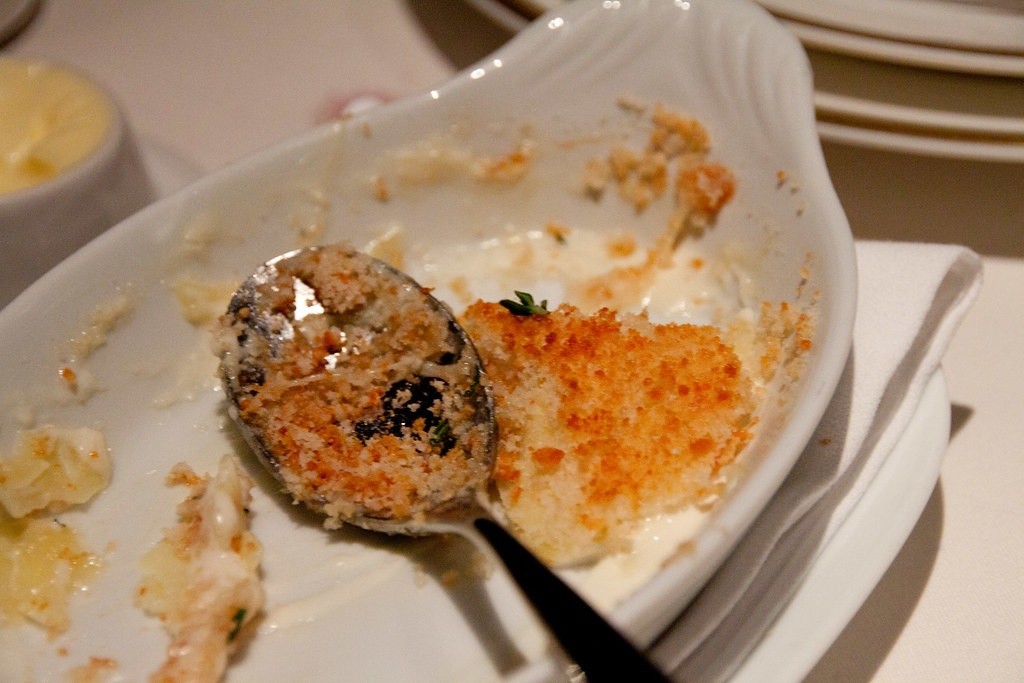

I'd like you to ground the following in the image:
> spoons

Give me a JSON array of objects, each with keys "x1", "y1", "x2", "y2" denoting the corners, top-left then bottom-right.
[{"x1": 217, "y1": 244, "x2": 675, "y2": 683}]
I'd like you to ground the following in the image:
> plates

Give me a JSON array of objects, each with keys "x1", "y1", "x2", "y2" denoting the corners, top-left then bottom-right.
[
  {"x1": 474, "y1": 0, "x2": 1024, "y2": 165},
  {"x1": 0, "y1": 0, "x2": 861, "y2": 683}
]
